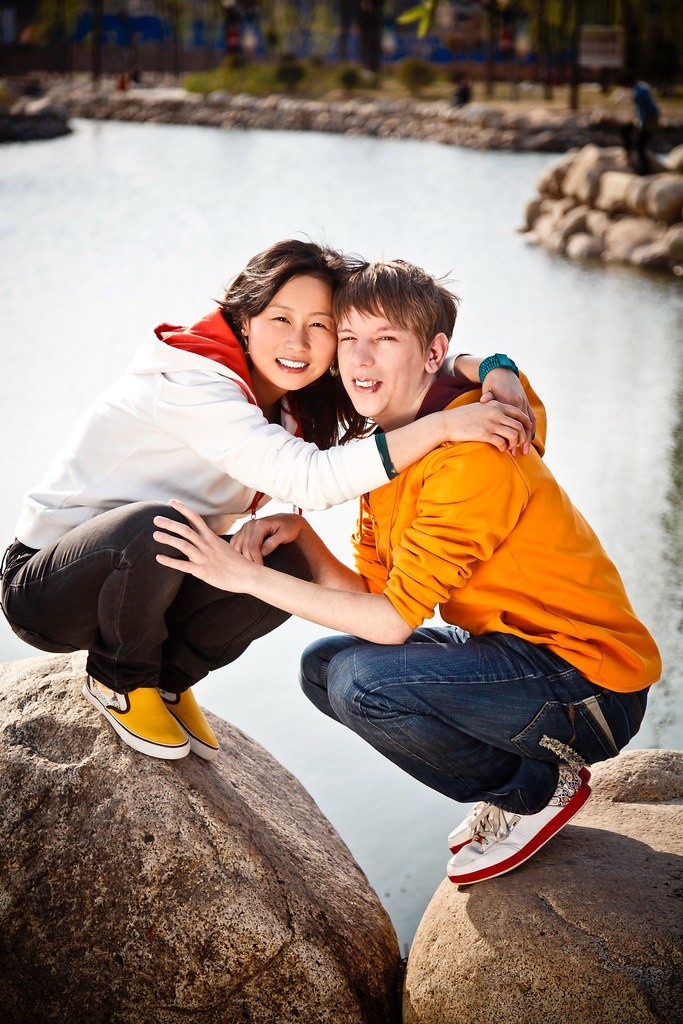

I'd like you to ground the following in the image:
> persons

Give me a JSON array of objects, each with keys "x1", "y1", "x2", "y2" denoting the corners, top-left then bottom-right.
[
  {"x1": 150, "y1": 257, "x2": 662, "y2": 887},
  {"x1": 0, "y1": 228, "x2": 534, "y2": 762},
  {"x1": 450, "y1": 79, "x2": 473, "y2": 110},
  {"x1": 115, "y1": 69, "x2": 130, "y2": 92},
  {"x1": 618, "y1": 71, "x2": 662, "y2": 177}
]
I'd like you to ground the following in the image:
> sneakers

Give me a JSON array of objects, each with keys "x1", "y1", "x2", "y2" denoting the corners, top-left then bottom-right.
[
  {"x1": 446, "y1": 766, "x2": 591, "y2": 885},
  {"x1": 156, "y1": 685, "x2": 220, "y2": 761},
  {"x1": 83, "y1": 673, "x2": 190, "y2": 760},
  {"x1": 449, "y1": 765, "x2": 592, "y2": 854}
]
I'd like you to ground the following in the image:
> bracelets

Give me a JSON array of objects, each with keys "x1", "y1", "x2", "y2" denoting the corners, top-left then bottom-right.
[{"x1": 478, "y1": 353, "x2": 520, "y2": 383}]
